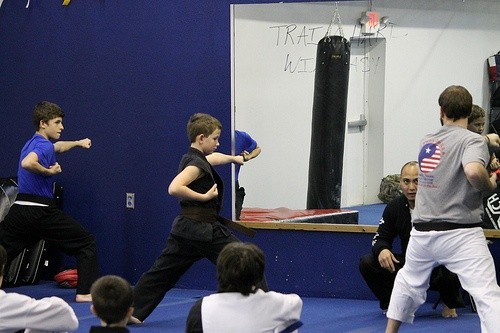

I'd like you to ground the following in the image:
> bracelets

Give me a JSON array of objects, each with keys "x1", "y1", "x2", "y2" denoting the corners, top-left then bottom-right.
[{"x1": 485, "y1": 134, "x2": 490, "y2": 145}]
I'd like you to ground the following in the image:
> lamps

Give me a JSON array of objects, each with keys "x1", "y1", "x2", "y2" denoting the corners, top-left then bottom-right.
[{"x1": 361, "y1": 14, "x2": 390, "y2": 27}]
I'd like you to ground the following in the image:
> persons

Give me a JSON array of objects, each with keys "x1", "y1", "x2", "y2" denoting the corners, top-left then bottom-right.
[
  {"x1": 385, "y1": 85, "x2": 500, "y2": 333},
  {"x1": 468, "y1": 105, "x2": 500, "y2": 169},
  {"x1": 87, "y1": 275, "x2": 135, "y2": 333},
  {"x1": 235, "y1": 130, "x2": 261, "y2": 221},
  {"x1": 359, "y1": 161, "x2": 467, "y2": 318},
  {"x1": 0, "y1": 245, "x2": 79, "y2": 333},
  {"x1": 0, "y1": 100, "x2": 99, "y2": 302},
  {"x1": 127, "y1": 113, "x2": 269, "y2": 324},
  {"x1": 186, "y1": 242, "x2": 303, "y2": 333}
]
